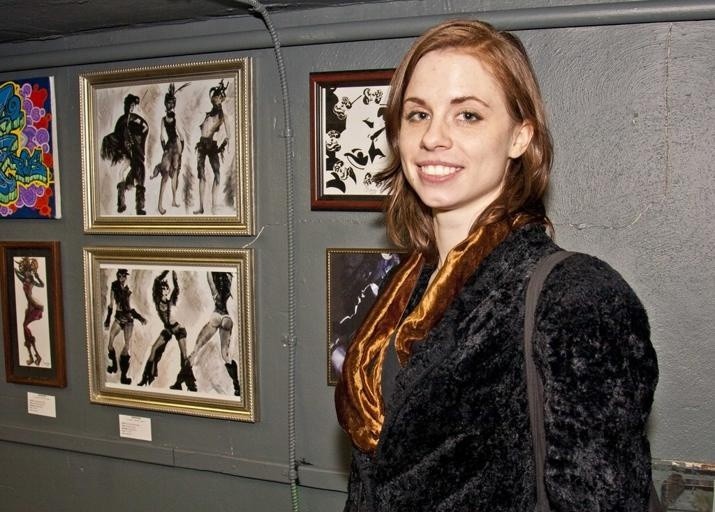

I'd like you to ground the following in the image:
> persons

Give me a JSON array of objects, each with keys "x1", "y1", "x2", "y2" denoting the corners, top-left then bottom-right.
[
  {"x1": 137, "y1": 269, "x2": 198, "y2": 392},
  {"x1": 193, "y1": 89, "x2": 232, "y2": 216},
  {"x1": 170, "y1": 272, "x2": 242, "y2": 397},
  {"x1": 150, "y1": 92, "x2": 184, "y2": 215},
  {"x1": 334, "y1": 18, "x2": 659, "y2": 511},
  {"x1": 15, "y1": 258, "x2": 45, "y2": 365},
  {"x1": 101, "y1": 93, "x2": 150, "y2": 215},
  {"x1": 103, "y1": 269, "x2": 147, "y2": 385}
]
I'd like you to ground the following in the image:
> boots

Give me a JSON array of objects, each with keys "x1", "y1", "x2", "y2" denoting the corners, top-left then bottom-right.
[{"x1": 106, "y1": 350, "x2": 241, "y2": 397}]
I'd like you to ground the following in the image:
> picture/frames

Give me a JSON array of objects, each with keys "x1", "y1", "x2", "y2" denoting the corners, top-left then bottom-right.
[
  {"x1": 0, "y1": 236, "x2": 69, "y2": 388},
  {"x1": 306, "y1": 67, "x2": 407, "y2": 214},
  {"x1": 323, "y1": 245, "x2": 414, "y2": 390},
  {"x1": 78, "y1": 53, "x2": 255, "y2": 239},
  {"x1": 78, "y1": 242, "x2": 257, "y2": 425}
]
